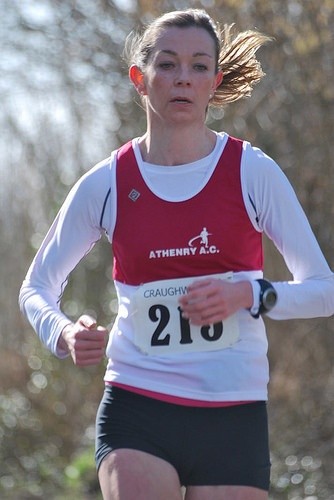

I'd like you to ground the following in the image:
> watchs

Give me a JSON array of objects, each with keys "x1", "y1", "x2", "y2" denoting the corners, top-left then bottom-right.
[{"x1": 251, "y1": 279, "x2": 277, "y2": 320}]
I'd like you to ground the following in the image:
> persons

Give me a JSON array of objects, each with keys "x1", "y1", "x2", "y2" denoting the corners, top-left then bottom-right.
[{"x1": 12, "y1": 9, "x2": 334, "y2": 499}]
